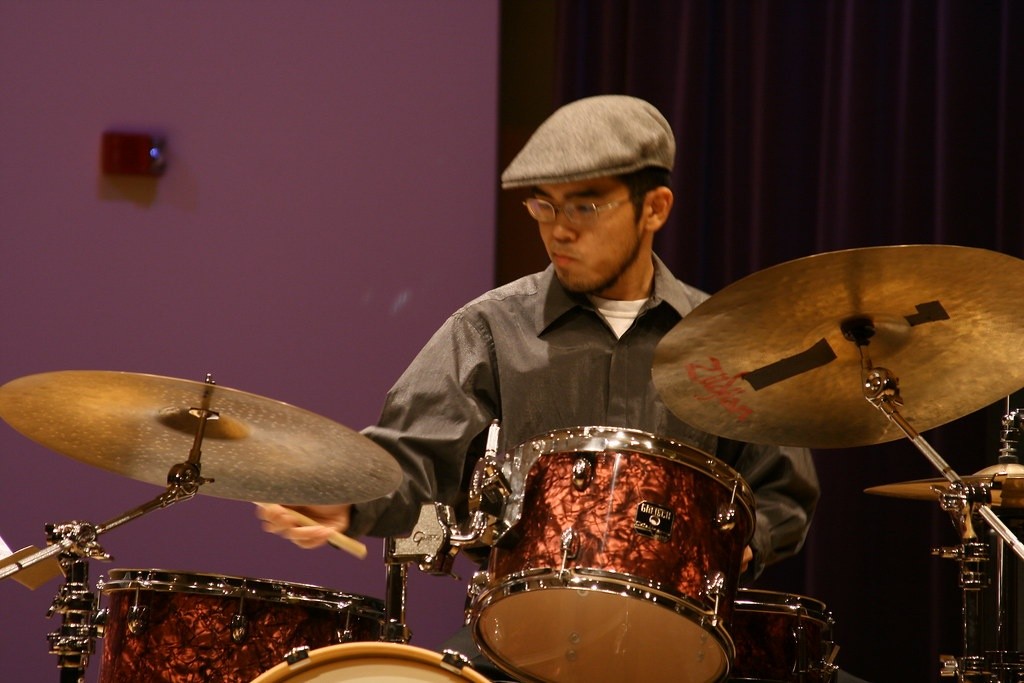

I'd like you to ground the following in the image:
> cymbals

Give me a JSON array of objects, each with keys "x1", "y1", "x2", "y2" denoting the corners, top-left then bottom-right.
[
  {"x1": 0, "y1": 365, "x2": 406, "y2": 508},
  {"x1": 648, "y1": 242, "x2": 1023, "y2": 447},
  {"x1": 864, "y1": 463, "x2": 1024, "y2": 511}
]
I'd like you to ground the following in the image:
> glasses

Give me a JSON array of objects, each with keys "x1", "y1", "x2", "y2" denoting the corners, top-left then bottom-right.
[{"x1": 520, "y1": 187, "x2": 648, "y2": 227}]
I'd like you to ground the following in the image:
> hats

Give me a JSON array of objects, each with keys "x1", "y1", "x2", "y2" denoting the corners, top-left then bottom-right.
[{"x1": 501, "y1": 95, "x2": 676, "y2": 189}]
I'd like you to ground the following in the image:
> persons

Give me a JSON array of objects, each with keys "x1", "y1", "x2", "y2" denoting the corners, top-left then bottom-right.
[{"x1": 258, "y1": 95, "x2": 819, "y2": 681}]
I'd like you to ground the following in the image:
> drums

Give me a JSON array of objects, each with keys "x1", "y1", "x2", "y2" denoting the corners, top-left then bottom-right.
[
  {"x1": 97, "y1": 568, "x2": 411, "y2": 683},
  {"x1": 243, "y1": 641, "x2": 490, "y2": 683},
  {"x1": 468, "y1": 421, "x2": 756, "y2": 683},
  {"x1": 731, "y1": 586, "x2": 836, "y2": 682}
]
